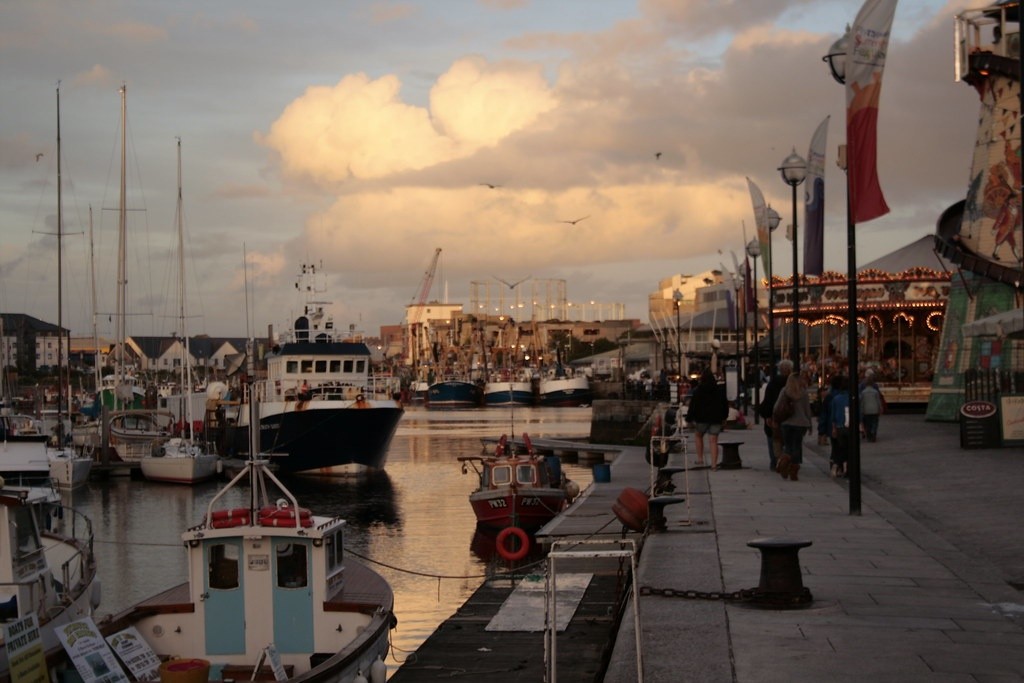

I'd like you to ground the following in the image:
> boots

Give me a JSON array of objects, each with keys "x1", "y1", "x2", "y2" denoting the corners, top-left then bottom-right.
[
  {"x1": 780, "y1": 452, "x2": 791, "y2": 479},
  {"x1": 789, "y1": 464, "x2": 800, "y2": 480},
  {"x1": 818, "y1": 433, "x2": 829, "y2": 446}
]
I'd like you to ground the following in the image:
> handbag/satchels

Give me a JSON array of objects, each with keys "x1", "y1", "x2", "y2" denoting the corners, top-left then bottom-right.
[{"x1": 775, "y1": 391, "x2": 796, "y2": 423}]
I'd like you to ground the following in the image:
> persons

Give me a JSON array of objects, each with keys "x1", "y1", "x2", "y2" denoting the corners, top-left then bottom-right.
[
  {"x1": 756, "y1": 360, "x2": 811, "y2": 470},
  {"x1": 813, "y1": 368, "x2": 886, "y2": 478},
  {"x1": 214, "y1": 559, "x2": 237, "y2": 589},
  {"x1": 627, "y1": 369, "x2": 652, "y2": 397},
  {"x1": 686, "y1": 370, "x2": 729, "y2": 473}
]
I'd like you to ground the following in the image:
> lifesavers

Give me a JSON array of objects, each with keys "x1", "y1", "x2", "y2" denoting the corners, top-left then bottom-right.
[
  {"x1": 651, "y1": 413, "x2": 661, "y2": 436},
  {"x1": 523, "y1": 432, "x2": 534, "y2": 456},
  {"x1": 259, "y1": 506, "x2": 314, "y2": 527},
  {"x1": 495, "y1": 434, "x2": 507, "y2": 458},
  {"x1": 203, "y1": 508, "x2": 250, "y2": 529},
  {"x1": 496, "y1": 526, "x2": 529, "y2": 560}
]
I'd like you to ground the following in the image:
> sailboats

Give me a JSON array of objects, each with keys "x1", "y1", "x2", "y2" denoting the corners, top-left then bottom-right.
[{"x1": 0, "y1": 80, "x2": 591, "y2": 498}]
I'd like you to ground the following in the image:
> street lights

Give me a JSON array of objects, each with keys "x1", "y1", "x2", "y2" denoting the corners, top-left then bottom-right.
[
  {"x1": 733, "y1": 275, "x2": 743, "y2": 411},
  {"x1": 746, "y1": 235, "x2": 762, "y2": 425},
  {"x1": 766, "y1": 202, "x2": 784, "y2": 374},
  {"x1": 820, "y1": 21, "x2": 864, "y2": 517},
  {"x1": 775, "y1": 145, "x2": 809, "y2": 376},
  {"x1": 672, "y1": 290, "x2": 684, "y2": 405}
]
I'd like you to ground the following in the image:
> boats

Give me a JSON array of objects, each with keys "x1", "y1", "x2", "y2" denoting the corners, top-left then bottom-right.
[
  {"x1": 42, "y1": 241, "x2": 398, "y2": 683},
  {"x1": 467, "y1": 355, "x2": 579, "y2": 535},
  {"x1": 0, "y1": 473, "x2": 102, "y2": 683}
]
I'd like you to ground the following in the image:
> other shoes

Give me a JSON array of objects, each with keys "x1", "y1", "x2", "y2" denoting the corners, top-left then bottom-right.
[
  {"x1": 694, "y1": 461, "x2": 703, "y2": 464},
  {"x1": 710, "y1": 466, "x2": 716, "y2": 471},
  {"x1": 871, "y1": 432, "x2": 876, "y2": 442}
]
